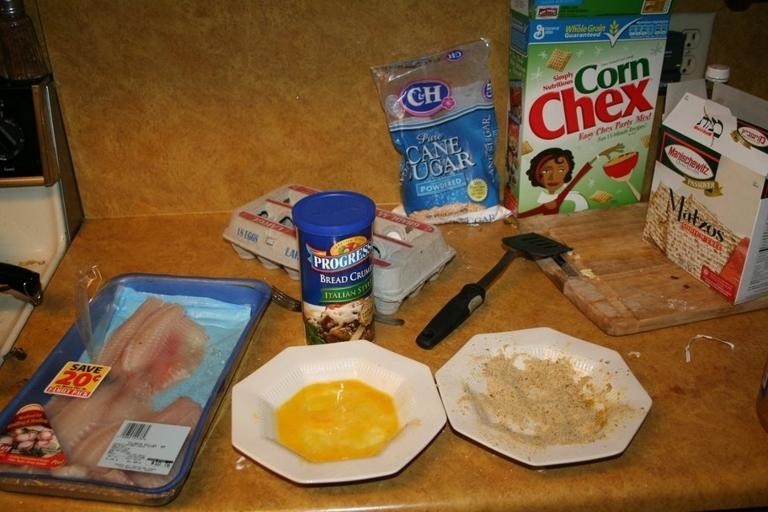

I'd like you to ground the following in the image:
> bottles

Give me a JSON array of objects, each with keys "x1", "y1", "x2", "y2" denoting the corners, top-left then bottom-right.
[{"x1": 703, "y1": 64, "x2": 731, "y2": 100}]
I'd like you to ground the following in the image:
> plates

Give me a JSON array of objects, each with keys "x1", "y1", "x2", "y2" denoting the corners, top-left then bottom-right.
[
  {"x1": 231, "y1": 339, "x2": 447, "y2": 484},
  {"x1": 435, "y1": 327, "x2": 653, "y2": 467}
]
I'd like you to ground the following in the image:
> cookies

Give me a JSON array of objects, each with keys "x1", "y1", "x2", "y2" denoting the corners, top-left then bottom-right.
[{"x1": 545, "y1": 49, "x2": 572, "y2": 73}]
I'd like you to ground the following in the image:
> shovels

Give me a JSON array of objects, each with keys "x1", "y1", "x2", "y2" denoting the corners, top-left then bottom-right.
[{"x1": 415, "y1": 231, "x2": 573, "y2": 350}]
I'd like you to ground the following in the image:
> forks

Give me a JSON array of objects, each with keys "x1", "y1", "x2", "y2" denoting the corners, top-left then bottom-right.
[{"x1": 269, "y1": 284, "x2": 405, "y2": 325}]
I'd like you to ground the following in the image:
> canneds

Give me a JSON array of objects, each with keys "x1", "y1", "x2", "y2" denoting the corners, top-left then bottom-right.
[{"x1": 291, "y1": 193, "x2": 376, "y2": 346}]
